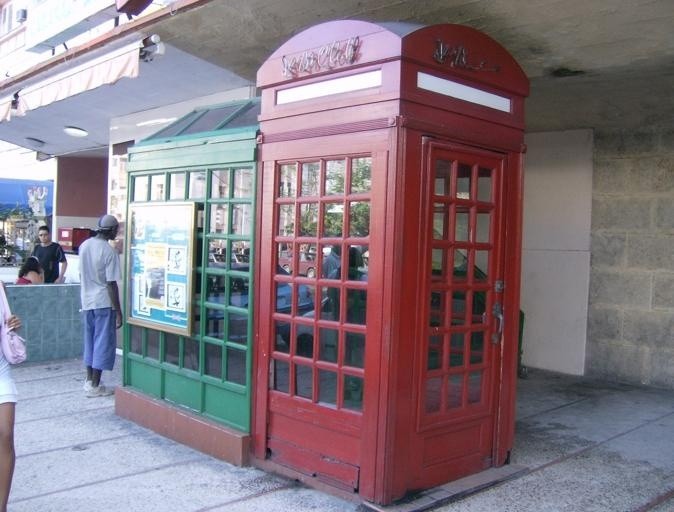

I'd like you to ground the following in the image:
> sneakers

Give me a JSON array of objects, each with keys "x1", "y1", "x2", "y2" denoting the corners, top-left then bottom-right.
[
  {"x1": 87, "y1": 383, "x2": 114, "y2": 398},
  {"x1": 84, "y1": 378, "x2": 92, "y2": 391}
]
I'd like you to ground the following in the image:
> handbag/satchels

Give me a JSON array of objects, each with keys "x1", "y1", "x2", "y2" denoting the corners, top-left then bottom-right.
[{"x1": 0, "y1": 313, "x2": 29, "y2": 366}]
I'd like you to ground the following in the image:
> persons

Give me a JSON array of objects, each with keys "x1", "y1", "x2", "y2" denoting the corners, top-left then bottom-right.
[
  {"x1": 324, "y1": 247, "x2": 370, "y2": 403},
  {"x1": 77, "y1": 213, "x2": 124, "y2": 400},
  {"x1": 0, "y1": 279, "x2": 25, "y2": 512},
  {"x1": 16, "y1": 256, "x2": 42, "y2": 285},
  {"x1": 321, "y1": 231, "x2": 352, "y2": 306},
  {"x1": 30, "y1": 225, "x2": 68, "y2": 285}
]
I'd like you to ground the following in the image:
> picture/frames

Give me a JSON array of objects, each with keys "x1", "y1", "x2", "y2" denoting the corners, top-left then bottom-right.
[{"x1": 125, "y1": 201, "x2": 199, "y2": 337}]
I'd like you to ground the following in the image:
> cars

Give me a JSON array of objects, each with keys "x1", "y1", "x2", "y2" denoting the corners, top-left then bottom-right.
[{"x1": 190, "y1": 225, "x2": 491, "y2": 387}]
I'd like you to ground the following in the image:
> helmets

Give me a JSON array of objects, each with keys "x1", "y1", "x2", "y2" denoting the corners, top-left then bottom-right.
[{"x1": 97, "y1": 214, "x2": 119, "y2": 231}]
